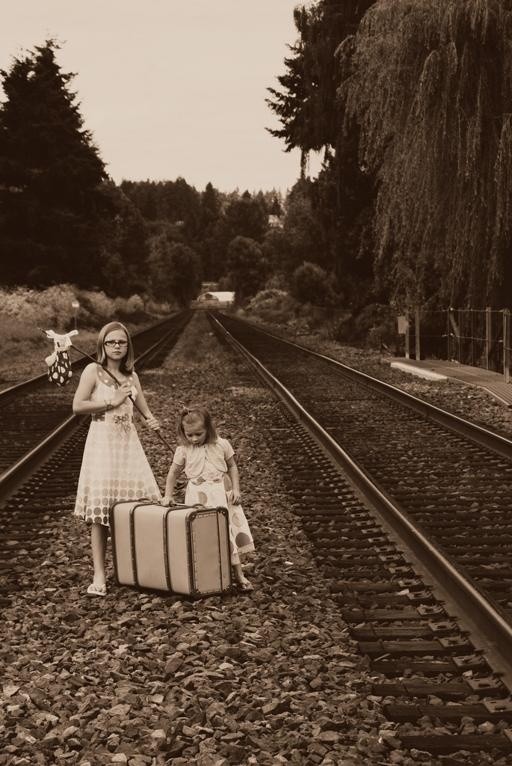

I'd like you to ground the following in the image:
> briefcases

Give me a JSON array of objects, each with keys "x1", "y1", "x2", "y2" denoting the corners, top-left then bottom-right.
[{"x1": 110, "y1": 498, "x2": 233, "y2": 599}]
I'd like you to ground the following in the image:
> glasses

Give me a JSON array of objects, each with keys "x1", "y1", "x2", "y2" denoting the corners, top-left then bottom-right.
[{"x1": 104, "y1": 341, "x2": 128, "y2": 347}]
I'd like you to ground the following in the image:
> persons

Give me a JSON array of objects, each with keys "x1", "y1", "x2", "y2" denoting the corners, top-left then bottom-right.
[
  {"x1": 70, "y1": 320, "x2": 165, "y2": 595},
  {"x1": 160, "y1": 406, "x2": 257, "y2": 592}
]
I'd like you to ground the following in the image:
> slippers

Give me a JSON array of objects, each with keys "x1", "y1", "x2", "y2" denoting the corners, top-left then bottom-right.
[
  {"x1": 232, "y1": 579, "x2": 254, "y2": 592},
  {"x1": 87, "y1": 583, "x2": 107, "y2": 597}
]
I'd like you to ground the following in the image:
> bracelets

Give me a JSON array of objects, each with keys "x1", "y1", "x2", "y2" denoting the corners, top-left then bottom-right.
[{"x1": 104, "y1": 400, "x2": 114, "y2": 409}]
